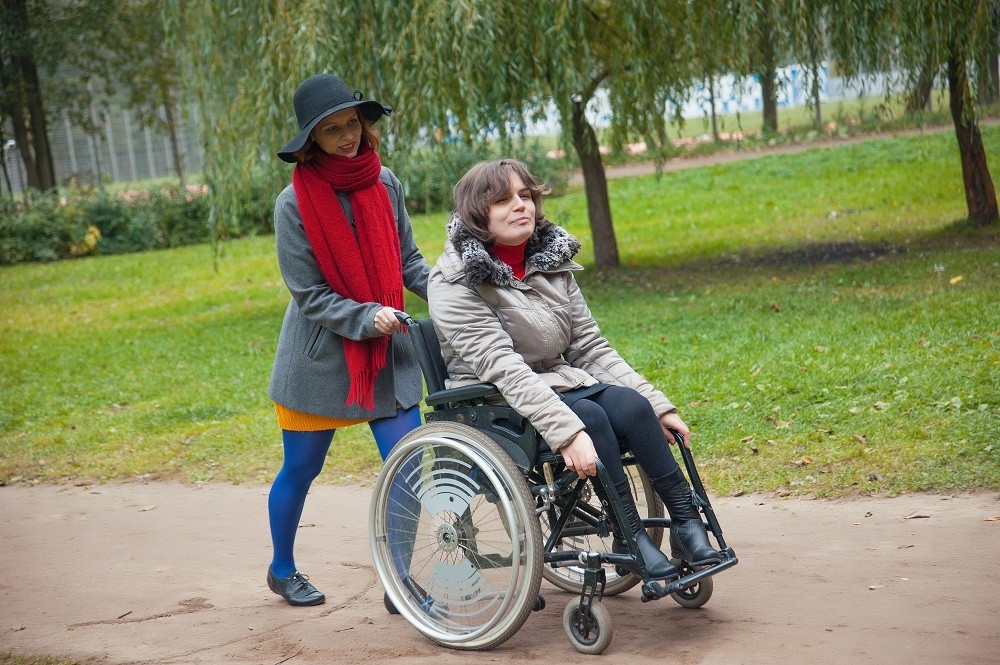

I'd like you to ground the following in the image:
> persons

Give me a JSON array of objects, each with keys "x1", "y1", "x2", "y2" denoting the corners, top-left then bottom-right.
[
  {"x1": 264, "y1": 74, "x2": 448, "y2": 622},
  {"x1": 425, "y1": 159, "x2": 725, "y2": 583}
]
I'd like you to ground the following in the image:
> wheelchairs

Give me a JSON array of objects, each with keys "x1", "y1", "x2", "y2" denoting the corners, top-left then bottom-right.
[{"x1": 367, "y1": 306, "x2": 741, "y2": 656}]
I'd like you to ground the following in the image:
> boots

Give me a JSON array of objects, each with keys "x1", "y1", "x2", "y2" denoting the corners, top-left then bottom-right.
[
  {"x1": 594, "y1": 475, "x2": 679, "y2": 578},
  {"x1": 648, "y1": 464, "x2": 721, "y2": 565}
]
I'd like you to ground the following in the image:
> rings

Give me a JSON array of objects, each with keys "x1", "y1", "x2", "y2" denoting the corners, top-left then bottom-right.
[{"x1": 385, "y1": 320, "x2": 389, "y2": 326}]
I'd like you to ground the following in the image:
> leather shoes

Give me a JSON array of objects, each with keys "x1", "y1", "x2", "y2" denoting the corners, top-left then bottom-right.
[
  {"x1": 383, "y1": 590, "x2": 449, "y2": 618},
  {"x1": 266, "y1": 564, "x2": 325, "y2": 605}
]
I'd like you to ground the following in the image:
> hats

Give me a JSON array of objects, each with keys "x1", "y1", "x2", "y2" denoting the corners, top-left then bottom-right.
[{"x1": 276, "y1": 73, "x2": 393, "y2": 163}]
